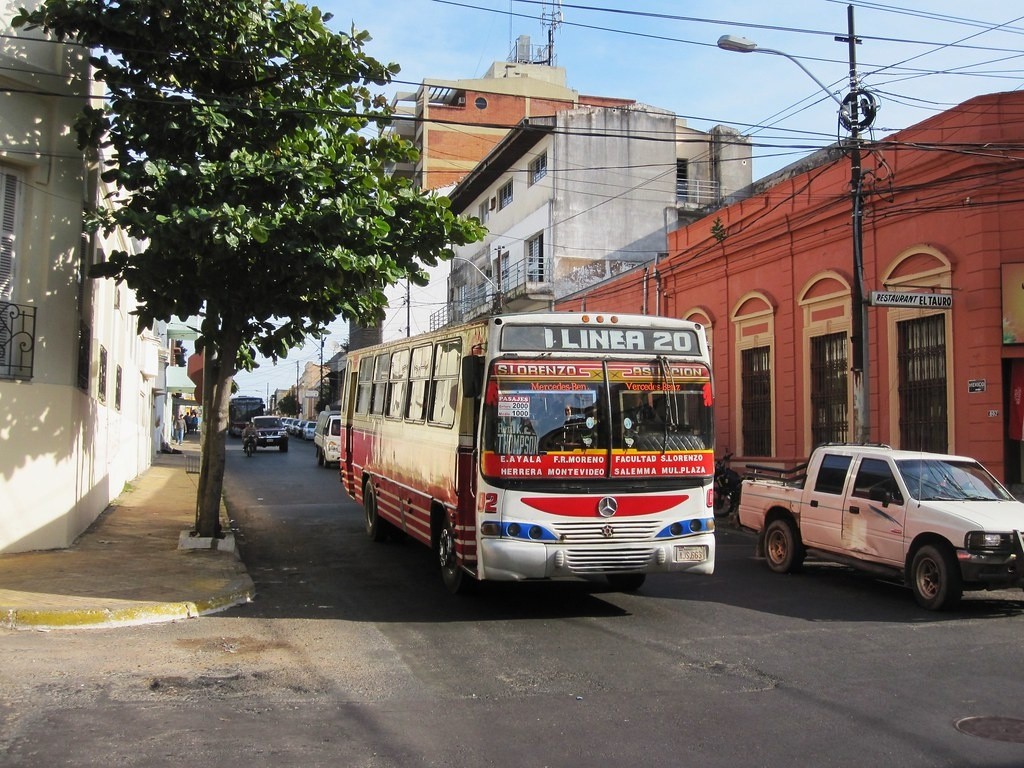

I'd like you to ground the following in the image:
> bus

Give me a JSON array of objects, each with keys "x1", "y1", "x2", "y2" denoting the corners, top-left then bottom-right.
[
  {"x1": 228, "y1": 396, "x2": 265, "y2": 438},
  {"x1": 340, "y1": 311, "x2": 714, "y2": 596}
]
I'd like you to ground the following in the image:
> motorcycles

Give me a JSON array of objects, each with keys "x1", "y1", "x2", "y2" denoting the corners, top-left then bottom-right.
[
  {"x1": 714, "y1": 448, "x2": 759, "y2": 517},
  {"x1": 244, "y1": 434, "x2": 255, "y2": 457}
]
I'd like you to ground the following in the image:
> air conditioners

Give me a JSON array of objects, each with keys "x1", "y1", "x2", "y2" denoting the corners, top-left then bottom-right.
[{"x1": 458, "y1": 97, "x2": 465, "y2": 106}]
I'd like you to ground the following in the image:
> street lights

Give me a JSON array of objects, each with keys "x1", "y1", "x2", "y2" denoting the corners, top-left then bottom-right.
[{"x1": 717, "y1": 34, "x2": 869, "y2": 447}]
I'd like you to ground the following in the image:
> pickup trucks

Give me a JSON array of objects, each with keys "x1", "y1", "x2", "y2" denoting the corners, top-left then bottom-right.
[{"x1": 738, "y1": 443, "x2": 1024, "y2": 610}]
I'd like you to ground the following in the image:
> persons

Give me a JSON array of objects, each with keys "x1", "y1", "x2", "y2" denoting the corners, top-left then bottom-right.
[
  {"x1": 623, "y1": 395, "x2": 664, "y2": 424},
  {"x1": 174, "y1": 409, "x2": 199, "y2": 445},
  {"x1": 241, "y1": 421, "x2": 259, "y2": 453},
  {"x1": 545, "y1": 397, "x2": 598, "y2": 442}
]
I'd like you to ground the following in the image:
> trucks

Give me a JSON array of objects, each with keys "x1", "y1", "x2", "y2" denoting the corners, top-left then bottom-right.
[{"x1": 314, "y1": 411, "x2": 341, "y2": 467}]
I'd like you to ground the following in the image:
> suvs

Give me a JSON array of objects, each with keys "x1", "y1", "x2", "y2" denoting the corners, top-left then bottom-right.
[{"x1": 250, "y1": 416, "x2": 288, "y2": 452}]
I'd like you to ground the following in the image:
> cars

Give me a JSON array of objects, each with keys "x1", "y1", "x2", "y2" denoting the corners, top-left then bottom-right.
[{"x1": 281, "y1": 417, "x2": 317, "y2": 440}]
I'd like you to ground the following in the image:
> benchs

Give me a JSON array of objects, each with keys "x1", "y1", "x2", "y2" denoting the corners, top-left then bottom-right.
[{"x1": 632, "y1": 432, "x2": 705, "y2": 450}]
